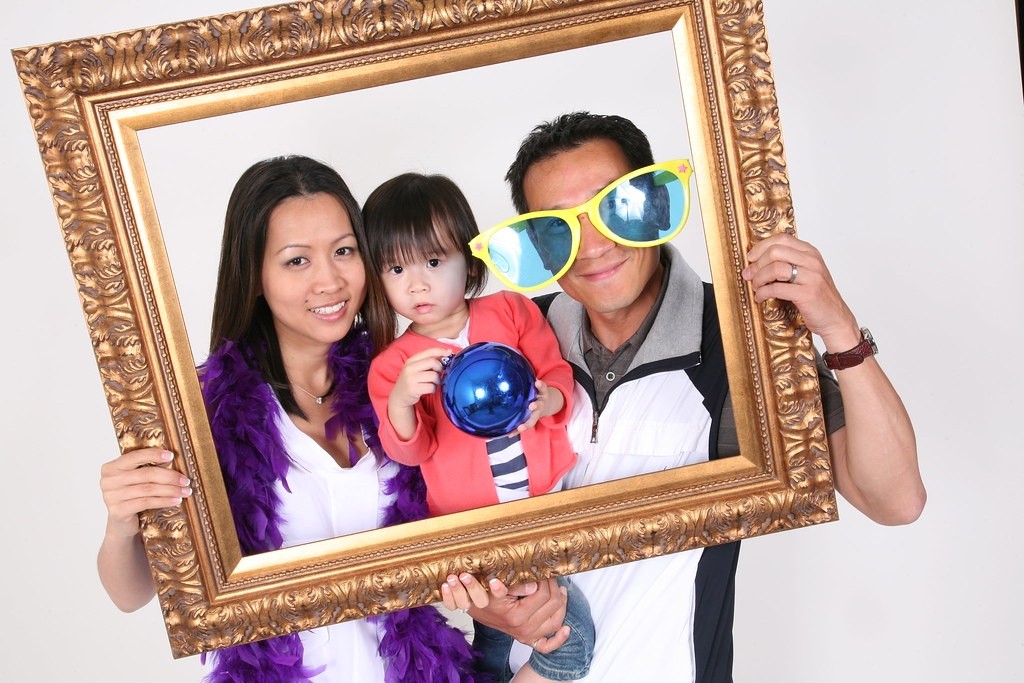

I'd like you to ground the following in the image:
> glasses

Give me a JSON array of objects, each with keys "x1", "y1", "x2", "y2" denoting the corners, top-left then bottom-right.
[{"x1": 468, "y1": 159, "x2": 695, "y2": 293}]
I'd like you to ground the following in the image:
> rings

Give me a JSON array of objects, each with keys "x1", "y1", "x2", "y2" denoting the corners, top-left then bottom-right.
[
  {"x1": 531, "y1": 639, "x2": 539, "y2": 650},
  {"x1": 457, "y1": 608, "x2": 469, "y2": 614},
  {"x1": 788, "y1": 263, "x2": 799, "y2": 284}
]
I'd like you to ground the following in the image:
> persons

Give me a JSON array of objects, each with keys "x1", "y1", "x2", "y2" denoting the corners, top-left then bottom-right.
[
  {"x1": 96, "y1": 155, "x2": 489, "y2": 683},
  {"x1": 357, "y1": 172, "x2": 597, "y2": 683},
  {"x1": 469, "y1": 108, "x2": 927, "y2": 683}
]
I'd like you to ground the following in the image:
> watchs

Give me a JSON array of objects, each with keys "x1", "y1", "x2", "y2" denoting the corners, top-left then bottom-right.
[{"x1": 822, "y1": 325, "x2": 879, "y2": 371}]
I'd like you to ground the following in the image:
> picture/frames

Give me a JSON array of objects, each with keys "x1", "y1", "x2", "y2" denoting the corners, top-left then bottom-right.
[{"x1": 11, "y1": 0, "x2": 839, "y2": 661}]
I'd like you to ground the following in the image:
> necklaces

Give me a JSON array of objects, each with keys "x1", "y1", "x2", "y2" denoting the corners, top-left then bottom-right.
[{"x1": 286, "y1": 377, "x2": 333, "y2": 405}]
